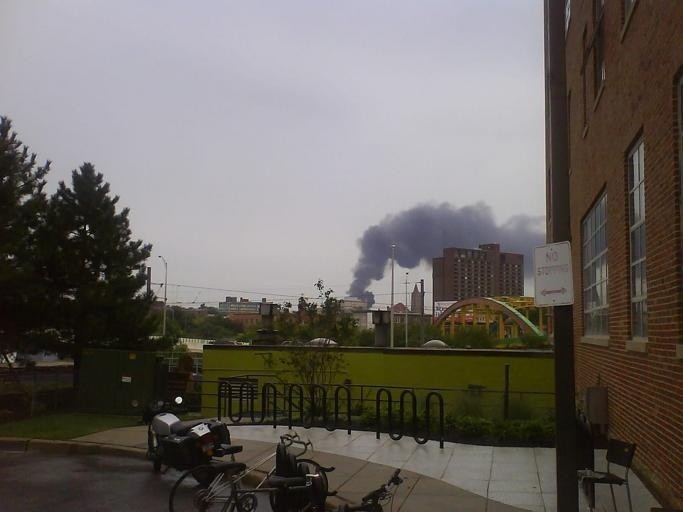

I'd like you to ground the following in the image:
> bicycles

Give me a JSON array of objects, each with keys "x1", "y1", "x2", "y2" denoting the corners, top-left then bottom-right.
[{"x1": 169, "y1": 429, "x2": 404, "y2": 512}]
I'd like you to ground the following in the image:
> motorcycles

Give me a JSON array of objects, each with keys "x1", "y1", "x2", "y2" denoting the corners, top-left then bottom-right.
[{"x1": 130, "y1": 396, "x2": 231, "y2": 485}]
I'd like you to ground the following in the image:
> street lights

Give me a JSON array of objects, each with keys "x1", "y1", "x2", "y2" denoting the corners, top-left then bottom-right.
[
  {"x1": 158, "y1": 254, "x2": 168, "y2": 333},
  {"x1": 390, "y1": 240, "x2": 397, "y2": 348},
  {"x1": 405, "y1": 272, "x2": 409, "y2": 347}
]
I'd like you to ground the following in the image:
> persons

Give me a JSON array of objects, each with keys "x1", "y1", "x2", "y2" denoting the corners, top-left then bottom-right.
[{"x1": 136, "y1": 352, "x2": 201, "y2": 425}]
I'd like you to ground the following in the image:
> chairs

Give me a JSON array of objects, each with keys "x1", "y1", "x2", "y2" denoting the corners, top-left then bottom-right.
[{"x1": 573, "y1": 438, "x2": 637, "y2": 512}]
